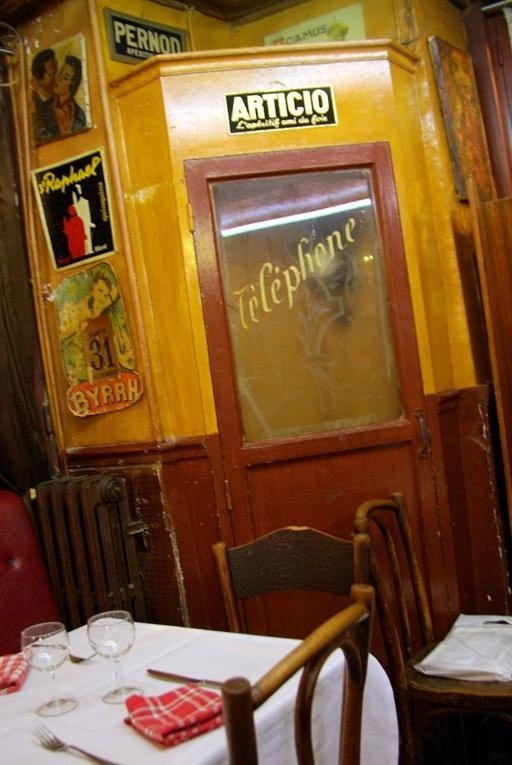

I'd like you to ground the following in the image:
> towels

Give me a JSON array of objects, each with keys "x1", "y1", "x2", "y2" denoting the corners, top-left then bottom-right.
[
  {"x1": 0, "y1": 656, "x2": 29, "y2": 695},
  {"x1": 124, "y1": 684, "x2": 224, "y2": 748}
]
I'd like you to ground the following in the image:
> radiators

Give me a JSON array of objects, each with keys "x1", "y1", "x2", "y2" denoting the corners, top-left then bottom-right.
[{"x1": 34, "y1": 475, "x2": 146, "y2": 630}]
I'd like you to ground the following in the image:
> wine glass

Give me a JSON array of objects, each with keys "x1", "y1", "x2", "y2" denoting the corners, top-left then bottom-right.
[
  {"x1": 87, "y1": 610, "x2": 143, "y2": 704},
  {"x1": 21, "y1": 621, "x2": 80, "y2": 717}
]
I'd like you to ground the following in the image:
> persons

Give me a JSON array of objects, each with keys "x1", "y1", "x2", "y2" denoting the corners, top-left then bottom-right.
[
  {"x1": 38, "y1": 55, "x2": 85, "y2": 139},
  {"x1": 31, "y1": 49, "x2": 58, "y2": 145},
  {"x1": 58, "y1": 271, "x2": 120, "y2": 342}
]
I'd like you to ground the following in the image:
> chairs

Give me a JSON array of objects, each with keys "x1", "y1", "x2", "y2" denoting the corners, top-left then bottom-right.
[
  {"x1": 354, "y1": 491, "x2": 512, "y2": 763},
  {"x1": 220, "y1": 583, "x2": 376, "y2": 765},
  {"x1": 1, "y1": 491, "x2": 63, "y2": 660},
  {"x1": 211, "y1": 526, "x2": 371, "y2": 648}
]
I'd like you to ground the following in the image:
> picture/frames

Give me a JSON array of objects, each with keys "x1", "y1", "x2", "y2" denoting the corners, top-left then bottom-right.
[
  {"x1": 31, "y1": 147, "x2": 118, "y2": 271},
  {"x1": 27, "y1": 32, "x2": 92, "y2": 147},
  {"x1": 428, "y1": 35, "x2": 503, "y2": 204}
]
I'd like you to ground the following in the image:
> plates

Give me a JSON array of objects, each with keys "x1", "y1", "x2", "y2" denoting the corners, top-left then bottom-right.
[{"x1": 147, "y1": 631, "x2": 304, "y2": 686}]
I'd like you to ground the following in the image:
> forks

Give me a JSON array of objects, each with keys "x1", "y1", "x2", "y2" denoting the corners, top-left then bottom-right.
[{"x1": 32, "y1": 723, "x2": 120, "y2": 765}]
